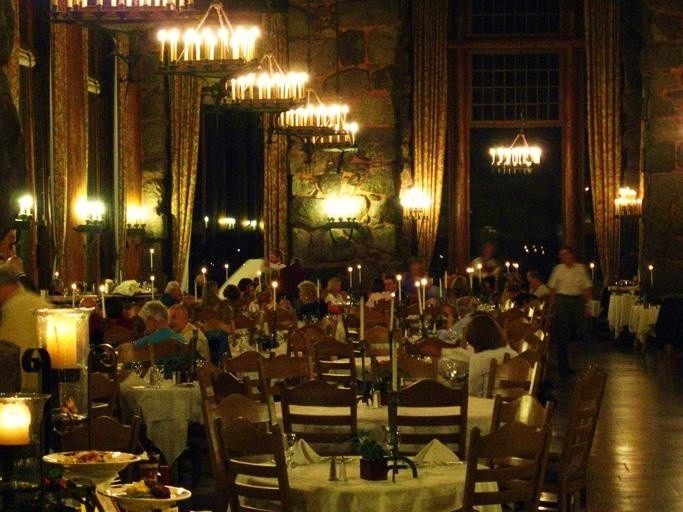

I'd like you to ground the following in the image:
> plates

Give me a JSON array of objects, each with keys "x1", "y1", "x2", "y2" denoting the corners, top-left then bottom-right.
[
  {"x1": 39, "y1": 450, "x2": 141, "y2": 480},
  {"x1": 97, "y1": 480, "x2": 192, "y2": 509}
]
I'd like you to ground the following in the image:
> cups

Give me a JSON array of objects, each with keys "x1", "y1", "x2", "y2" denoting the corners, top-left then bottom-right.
[{"x1": 444, "y1": 361, "x2": 456, "y2": 380}]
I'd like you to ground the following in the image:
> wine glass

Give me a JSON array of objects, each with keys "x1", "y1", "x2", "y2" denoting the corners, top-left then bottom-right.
[
  {"x1": 272, "y1": 433, "x2": 298, "y2": 472},
  {"x1": 151, "y1": 364, "x2": 165, "y2": 389}
]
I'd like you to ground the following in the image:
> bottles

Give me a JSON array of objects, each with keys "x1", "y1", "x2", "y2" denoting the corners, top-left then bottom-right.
[{"x1": 328, "y1": 453, "x2": 348, "y2": 482}]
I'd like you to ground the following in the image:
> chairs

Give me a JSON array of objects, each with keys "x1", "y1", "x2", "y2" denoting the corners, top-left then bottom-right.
[{"x1": 640, "y1": 295, "x2": 682, "y2": 357}]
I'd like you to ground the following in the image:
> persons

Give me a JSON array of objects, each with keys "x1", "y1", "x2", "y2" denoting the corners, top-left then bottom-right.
[{"x1": 1, "y1": 240, "x2": 596, "y2": 392}]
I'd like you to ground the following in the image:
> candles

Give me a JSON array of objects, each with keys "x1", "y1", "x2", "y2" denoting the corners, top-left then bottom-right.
[{"x1": 0, "y1": 313, "x2": 77, "y2": 443}]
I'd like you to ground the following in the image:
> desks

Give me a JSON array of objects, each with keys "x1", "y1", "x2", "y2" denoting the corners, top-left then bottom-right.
[{"x1": 607, "y1": 291, "x2": 660, "y2": 363}]
